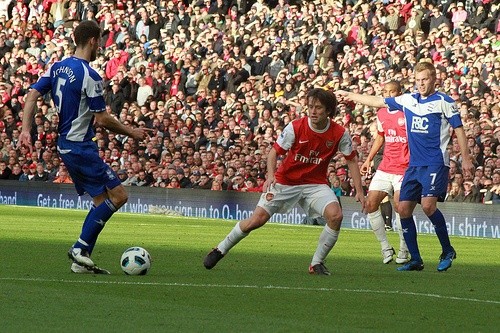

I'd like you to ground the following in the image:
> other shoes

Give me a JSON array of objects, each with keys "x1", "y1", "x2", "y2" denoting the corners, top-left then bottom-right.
[{"x1": 384, "y1": 224, "x2": 393, "y2": 232}]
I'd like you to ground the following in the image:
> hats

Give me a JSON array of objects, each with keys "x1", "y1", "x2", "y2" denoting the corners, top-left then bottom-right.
[{"x1": 0, "y1": 0, "x2": 499, "y2": 182}]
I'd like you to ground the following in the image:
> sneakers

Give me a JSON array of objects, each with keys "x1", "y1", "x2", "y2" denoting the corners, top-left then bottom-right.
[
  {"x1": 70, "y1": 259, "x2": 111, "y2": 274},
  {"x1": 437, "y1": 245, "x2": 456, "y2": 271},
  {"x1": 381, "y1": 245, "x2": 395, "y2": 264},
  {"x1": 395, "y1": 247, "x2": 410, "y2": 263},
  {"x1": 204, "y1": 247, "x2": 225, "y2": 269},
  {"x1": 67, "y1": 243, "x2": 95, "y2": 267},
  {"x1": 309, "y1": 262, "x2": 333, "y2": 276},
  {"x1": 397, "y1": 257, "x2": 425, "y2": 271}
]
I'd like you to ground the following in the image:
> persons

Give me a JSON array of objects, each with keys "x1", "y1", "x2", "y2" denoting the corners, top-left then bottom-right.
[
  {"x1": 0, "y1": 0, "x2": 500, "y2": 275},
  {"x1": 15, "y1": 20, "x2": 150, "y2": 274}
]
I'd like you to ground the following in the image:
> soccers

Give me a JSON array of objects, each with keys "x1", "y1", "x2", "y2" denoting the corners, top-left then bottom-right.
[{"x1": 120, "y1": 246, "x2": 152, "y2": 276}]
